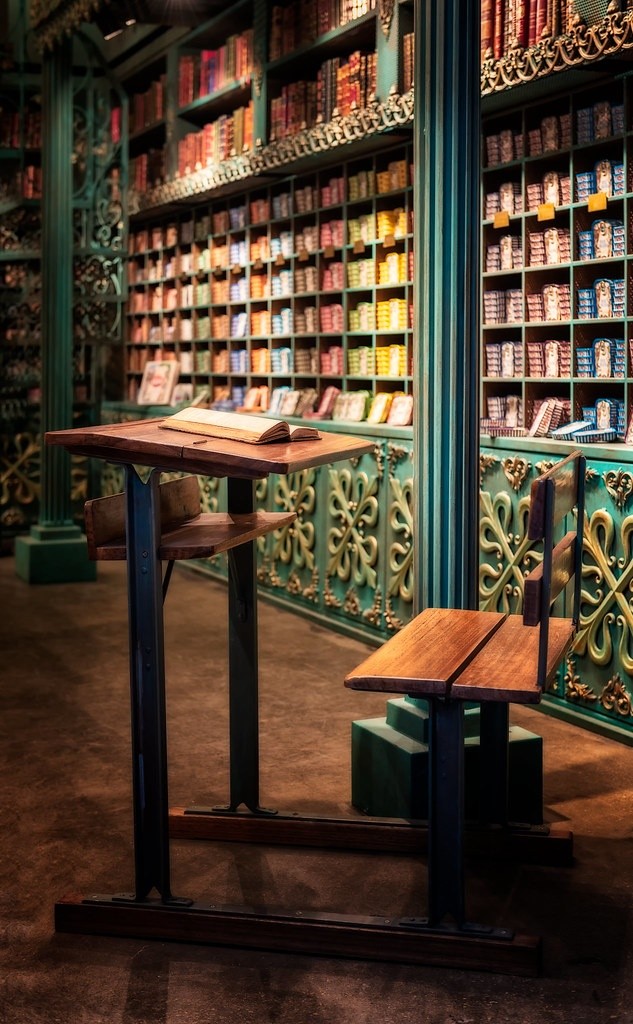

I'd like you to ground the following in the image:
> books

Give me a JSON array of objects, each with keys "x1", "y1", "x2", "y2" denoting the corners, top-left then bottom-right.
[
  {"x1": 176, "y1": 100, "x2": 256, "y2": 182},
  {"x1": 109, "y1": 145, "x2": 168, "y2": 202},
  {"x1": 269, "y1": 0, "x2": 382, "y2": 66},
  {"x1": 178, "y1": 24, "x2": 253, "y2": 111},
  {"x1": 158, "y1": 405, "x2": 323, "y2": 445},
  {"x1": 481, "y1": 0, "x2": 584, "y2": 66},
  {"x1": 268, "y1": 33, "x2": 416, "y2": 143},
  {"x1": 106, "y1": 67, "x2": 169, "y2": 143},
  {"x1": 1, "y1": 95, "x2": 44, "y2": 199}
]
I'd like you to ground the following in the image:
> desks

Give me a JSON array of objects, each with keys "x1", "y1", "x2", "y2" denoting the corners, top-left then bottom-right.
[{"x1": 44, "y1": 416, "x2": 586, "y2": 978}]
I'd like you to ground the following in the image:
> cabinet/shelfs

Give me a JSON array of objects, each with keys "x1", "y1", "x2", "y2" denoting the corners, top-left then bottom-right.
[{"x1": 1, "y1": 1, "x2": 633, "y2": 748}]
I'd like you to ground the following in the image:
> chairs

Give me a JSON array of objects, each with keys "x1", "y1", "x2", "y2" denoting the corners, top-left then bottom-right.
[{"x1": 342, "y1": 449, "x2": 586, "y2": 943}]
